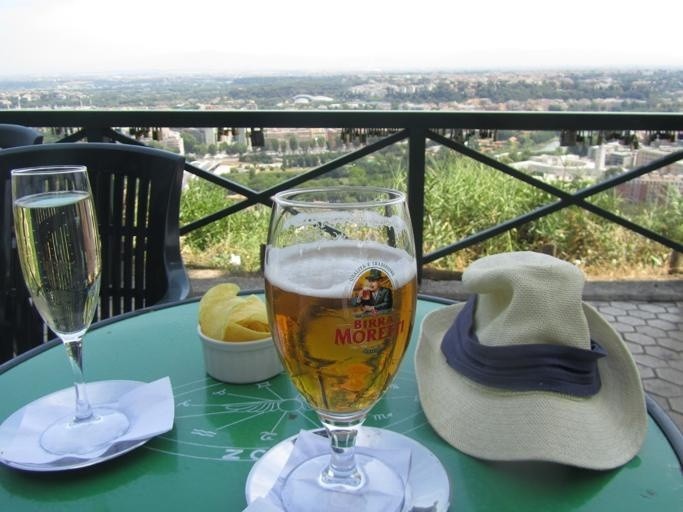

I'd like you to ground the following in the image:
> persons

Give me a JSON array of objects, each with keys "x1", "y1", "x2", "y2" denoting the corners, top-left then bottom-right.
[{"x1": 352, "y1": 268, "x2": 393, "y2": 312}]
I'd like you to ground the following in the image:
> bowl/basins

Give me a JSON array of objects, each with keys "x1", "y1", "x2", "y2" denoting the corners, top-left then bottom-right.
[{"x1": 198, "y1": 319, "x2": 288, "y2": 387}]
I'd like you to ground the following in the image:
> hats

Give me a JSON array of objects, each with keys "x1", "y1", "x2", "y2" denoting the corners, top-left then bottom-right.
[{"x1": 412, "y1": 250, "x2": 646, "y2": 472}]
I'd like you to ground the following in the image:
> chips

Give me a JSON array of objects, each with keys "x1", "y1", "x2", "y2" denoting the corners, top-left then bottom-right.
[{"x1": 199, "y1": 282, "x2": 272, "y2": 341}]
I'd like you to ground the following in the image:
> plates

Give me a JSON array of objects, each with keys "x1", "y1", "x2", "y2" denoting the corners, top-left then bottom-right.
[
  {"x1": 242, "y1": 425, "x2": 455, "y2": 510},
  {"x1": 1, "y1": 378, "x2": 159, "y2": 473}
]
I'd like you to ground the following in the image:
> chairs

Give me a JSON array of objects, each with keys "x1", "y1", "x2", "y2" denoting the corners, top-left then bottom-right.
[
  {"x1": 0, "y1": 142, "x2": 194, "y2": 366},
  {"x1": 0, "y1": 123, "x2": 43, "y2": 151}
]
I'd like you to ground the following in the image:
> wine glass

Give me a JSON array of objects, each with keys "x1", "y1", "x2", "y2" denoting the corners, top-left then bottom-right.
[
  {"x1": 267, "y1": 183, "x2": 417, "y2": 511},
  {"x1": 11, "y1": 164, "x2": 133, "y2": 455}
]
